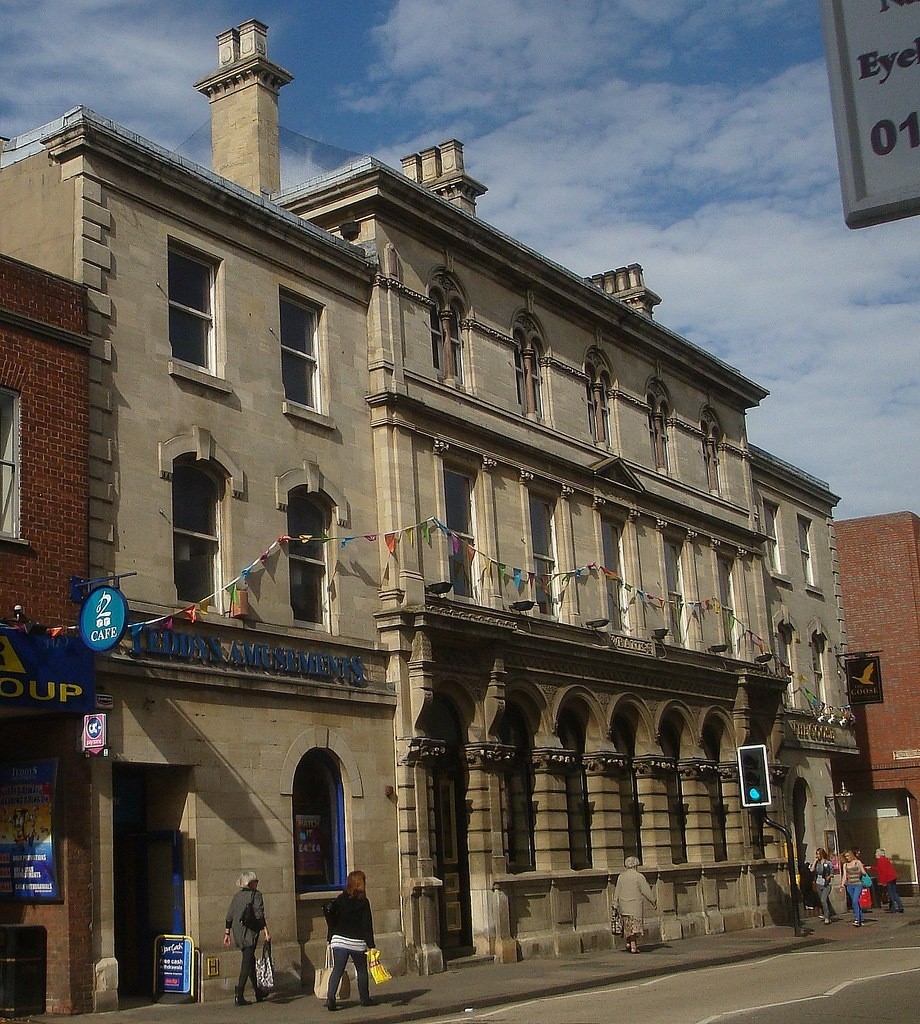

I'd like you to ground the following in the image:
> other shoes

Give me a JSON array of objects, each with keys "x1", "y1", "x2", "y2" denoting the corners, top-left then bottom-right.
[
  {"x1": 819, "y1": 915, "x2": 825, "y2": 919},
  {"x1": 360, "y1": 998, "x2": 378, "y2": 1005},
  {"x1": 824, "y1": 917, "x2": 831, "y2": 924},
  {"x1": 326, "y1": 999, "x2": 338, "y2": 1010},
  {"x1": 234, "y1": 996, "x2": 251, "y2": 1005},
  {"x1": 852, "y1": 920, "x2": 861, "y2": 927},
  {"x1": 254, "y1": 992, "x2": 270, "y2": 1002}
]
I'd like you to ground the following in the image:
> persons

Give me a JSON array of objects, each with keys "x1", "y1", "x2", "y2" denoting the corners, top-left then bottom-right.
[
  {"x1": 613, "y1": 856, "x2": 657, "y2": 954},
  {"x1": 840, "y1": 849, "x2": 867, "y2": 927},
  {"x1": 865, "y1": 849, "x2": 904, "y2": 914},
  {"x1": 223, "y1": 870, "x2": 271, "y2": 1008},
  {"x1": 811, "y1": 848, "x2": 834, "y2": 925},
  {"x1": 323, "y1": 870, "x2": 377, "y2": 1011}
]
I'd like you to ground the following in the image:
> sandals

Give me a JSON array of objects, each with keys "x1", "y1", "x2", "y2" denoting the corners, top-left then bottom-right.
[{"x1": 626, "y1": 944, "x2": 639, "y2": 953}]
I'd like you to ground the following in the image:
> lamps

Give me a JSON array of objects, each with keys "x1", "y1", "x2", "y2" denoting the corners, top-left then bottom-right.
[
  {"x1": 509, "y1": 599, "x2": 536, "y2": 613},
  {"x1": 810, "y1": 705, "x2": 847, "y2": 726},
  {"x1": 824, "y1": 781, "x2": 854, "y2": 814},
  {"x1": 709, "y1": 644, "x2": 729, "y2": 653},
  {"x1": 651, "y1": 628, "x2": 671, "y2": 641},
  {"x1": 587, "y1": 618, "x2": 611, "y2": 629},
  {"x1": 426, "y1": 580, "x2": 453, "y2": 596},
  {"x1": 756, "y1": 653, "x2": 775, "y2": 664}
]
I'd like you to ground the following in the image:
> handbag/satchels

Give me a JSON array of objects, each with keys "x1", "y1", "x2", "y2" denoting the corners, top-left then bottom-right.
[
  {"x1": 314, "y1": 946, "x2": 350, "y2": 998},
  {"x1": 611, "y1": 908, "x2": 623, "y2": 936},
  {"x1": 365, "y1": 947, "x2": 391, "y2": 984},
  {"x1": 319, "y1": 893, "x2": 345, "y2": 931},
  {"x1": 240, "y1": 890, "x2": 266, "y2": 929},
  {"x1": 861, "y1": 873, "x2": 872, "y2": 888},
  {"x1": 254, "y1": 938, "x2": 275, "y2": 994}
]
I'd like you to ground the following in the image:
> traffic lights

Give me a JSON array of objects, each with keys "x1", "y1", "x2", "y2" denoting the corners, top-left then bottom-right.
[{"x1": 737, "y1": 744, "x2": 772, "y2": 808}]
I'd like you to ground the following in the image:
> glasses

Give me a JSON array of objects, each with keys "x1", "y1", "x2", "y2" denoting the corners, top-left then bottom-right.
[{"x1": 251, "y1": 879, "x2": 259, "y2": 884}]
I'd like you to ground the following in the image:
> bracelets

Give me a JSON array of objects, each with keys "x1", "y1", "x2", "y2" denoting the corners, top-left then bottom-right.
[{"x1": 225, "y1": 932, "x2": 230, "y2": 936}]
